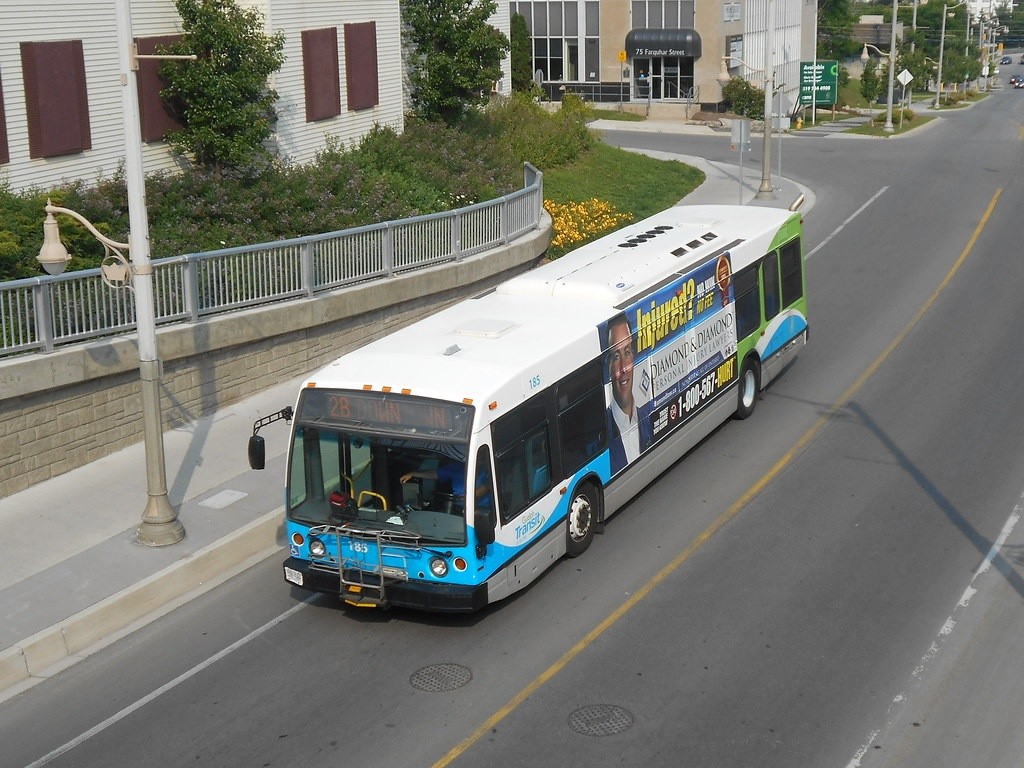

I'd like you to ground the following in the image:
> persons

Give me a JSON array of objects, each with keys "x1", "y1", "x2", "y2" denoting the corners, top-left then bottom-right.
[
  {"x1": 399, "y1": 462, "x2": 493, "y2": 514},
  {"x1": 606, "y1": 314, "x2": 649, "y2": 477}
]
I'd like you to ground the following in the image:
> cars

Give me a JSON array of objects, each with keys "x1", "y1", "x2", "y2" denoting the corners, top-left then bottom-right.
[
  {"x1": 1000, "y1": 56, "x2": 1012, "y2": 64},
  {"x1": 1020, "y1": 55, "x2": 1024, "y2": 64},
  {"x1": 1010, "y1": 74, "x2": 1021, "y2": 83},
  {"x1": 1014, "y1": 78, "x2": 1024, "y2": 89}
]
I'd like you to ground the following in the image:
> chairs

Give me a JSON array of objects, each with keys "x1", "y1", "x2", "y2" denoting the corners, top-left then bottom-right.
[
  {"x1": 733, "y1": 292, "x2": 777, "y2": 340},
  {"x1": 533, "y1": 437, "x2": 599, "y2": 499}
]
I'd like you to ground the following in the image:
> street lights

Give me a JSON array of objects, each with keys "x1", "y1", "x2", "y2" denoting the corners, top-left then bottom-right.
[
  {"x1": 977, "y1": 18, "x2": 1000, "y2": 91},
  {"x1": 861, "y1": 43, "x2": 895, "y2": 132},
  {"x1": 923, "y1": 2, "x2": 972, "y2": 109},
  {"x1": 36, "y1": 198, "x2": 187, "y2": 547},
  {"x1": 716, "y1": 55, "x2": 777, "y2": 200},
  {"x1": 983, "y1": 25, "x2": 1009, "y2": 92}
]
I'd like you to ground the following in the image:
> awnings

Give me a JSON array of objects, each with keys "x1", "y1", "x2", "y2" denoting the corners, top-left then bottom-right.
[{"x1": 625, "y1": 28, "x2": 702, "y2": 58}]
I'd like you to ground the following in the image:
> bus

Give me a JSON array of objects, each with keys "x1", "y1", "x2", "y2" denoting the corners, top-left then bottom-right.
[{"x1": 248, "y1": 205, "x2": 810, "y2": 614}]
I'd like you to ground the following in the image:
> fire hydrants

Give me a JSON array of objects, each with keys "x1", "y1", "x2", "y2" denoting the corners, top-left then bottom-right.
[{"x1": 796, "y1": 117, "x2": 803, "y2": 130}]
